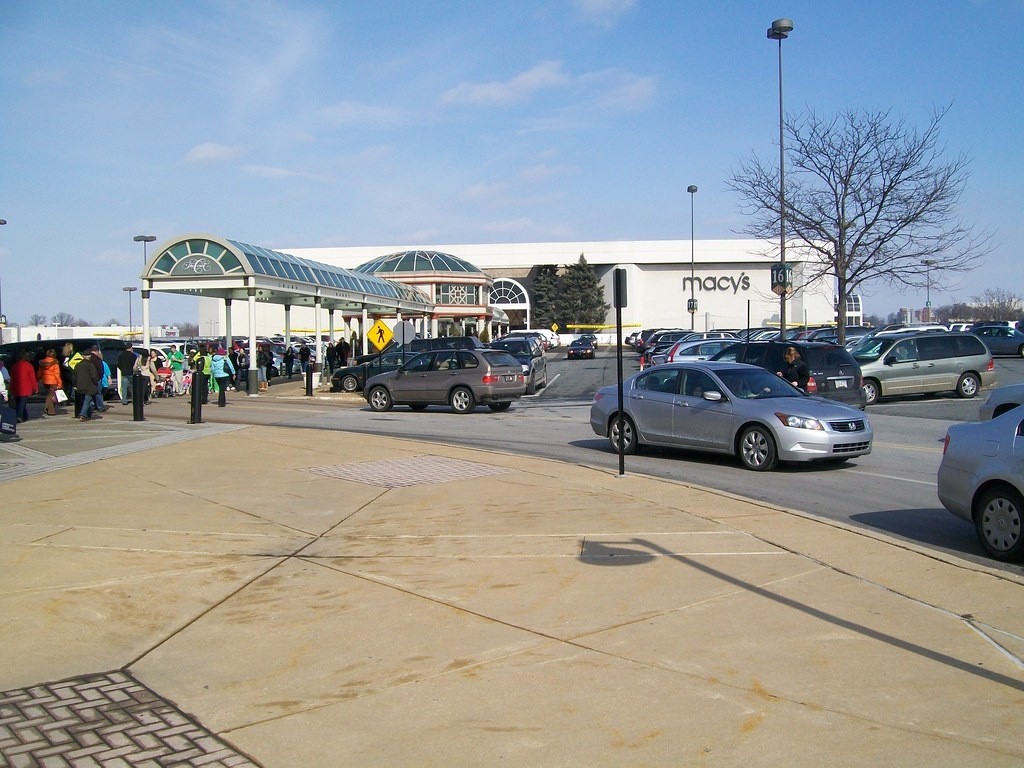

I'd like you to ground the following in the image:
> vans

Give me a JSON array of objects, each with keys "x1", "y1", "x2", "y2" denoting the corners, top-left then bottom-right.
[
  {"x1": 355, "y1": 336, "x2": 485, "y2": 368},
  {"x1": 0, "y1": 338, "x2": 125, "y2": 401},
  {"x1": 852, "y1": 333, "x2": 994, "y2": 404}
]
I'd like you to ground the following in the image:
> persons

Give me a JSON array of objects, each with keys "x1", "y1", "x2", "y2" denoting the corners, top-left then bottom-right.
[
  {"x1": 729, "y1": 374, "x2": 771, "y2": 398},
  {"x1": 299, "y1": 341, "x2": 311, "y2": 381},
  {"x1": 284, "y1": 346, "x2": 295, "y2": 380},
  {"x1": 777, "y1": 347, "x2": 810, "y2": 394},
  {"x1": 60, "y1": 342, "x2": 112, "y2": 422},
  {"x1": 182, "y1": 376, "x2": 192, "y2": 393},
  {"x1": 116, "y1": 342, "x2": 163, "y2": 405},
  {"x1": 0, "y1": 358, "x2": 11, "y2": 401},
  {"x1": 690, "y1": 383, "x2": 703, "y2": 397},
  {"x1": 188, "y1": 345, "x2": 250, "y2": 407},
  {"x1": 35, "y1": 348, "x2": 62, "y2": 418},
  {"x1": 325, "y1": 343, "x2": 335, "y2": 375},
  {"x1": 10, "y1": 350, "x2": 38, "y2": 422},
  {"x1": 335, "y1": 330, "x2": 365, "y2": 368},
  {"x1": 256, "y1": 344, "x2": 275, "y2": 391},
  {"x1": 428, "y1": 333, "x2": 432, "y2": 338},
  {"x1": 166, "y1": 344, "x2": 184, "y2": 395},
  {"x1": 418, "y1": 358, "x2": 441, "y2": 371}
]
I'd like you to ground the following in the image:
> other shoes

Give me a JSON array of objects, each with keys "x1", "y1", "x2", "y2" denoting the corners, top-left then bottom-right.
[
  {"x1": 227, "y1": 387, "x2": 236, "y2": 391},
  {"x1": 43, "y1": 407, "x2": 48, "y2": 414},
  {"x1": 219, "y1": 404, "x2": 225, "y2": 407},
  {"x1": 92, "y1": 416, "x2": 103, "y2": 419},
  {"x1": 80, "y1": 415, "x2": 88, "y2": 422},
  {"x1": 101, "y1": 405, "x2": 109, "y2": 412},
  {"x1": 17, "y1": 417, "x2": 23, "y2": 423}
]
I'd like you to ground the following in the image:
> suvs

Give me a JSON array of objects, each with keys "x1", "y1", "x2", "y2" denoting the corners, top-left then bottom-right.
[
  {"x1": 665, "y1": 341, "x2": 866, "y2": 412},
  {"x1": 487, "y1": 336, "x2": 547, "y2": 395},
  {"x1": 364, "y1": 348, "x2": 526, "y2": 415}
]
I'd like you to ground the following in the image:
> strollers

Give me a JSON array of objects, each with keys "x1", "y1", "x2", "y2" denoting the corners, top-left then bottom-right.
[{"x1": 151, "y1": 368, "x2": 175, "y2": 398}]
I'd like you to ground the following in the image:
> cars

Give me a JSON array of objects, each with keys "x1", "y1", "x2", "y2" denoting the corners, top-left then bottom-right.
[
  {"x1": 568, "y1": 340, "x2": 595, "y2": 359},
  {"x1": 936, "y1": 383, "x2": 1024, "y2": 565},
  {"x1": 625, "y1": 321, "x2": 1024, "y2": 370},
  {"x1": 124, "y1": 333, "x2": 335, "y2": 381},
  {"x1": 579, "y1": 334, "x2": 598, "y2": 349},
  {"x1": 331, "y1": 352, "x2": 429, "y2": 392},
  {"x1": 590, "y1": 359, "x2": 874, "y2": 470},
  {"x1": 495, "y1": 331, "x2": 554, "y2": 352}
]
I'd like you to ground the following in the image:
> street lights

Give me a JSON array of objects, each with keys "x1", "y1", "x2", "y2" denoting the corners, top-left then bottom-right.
[
  {"x1": 921, "y1": 259, "x2": 936, "y2": 322},
  {"x1": 134, "y1": 235, "x2": 156, "y2": 266},
  {"x1": 122, "y1": 287, "x2": 137, "y2": 341},
  {"x1": 767, "y1": 18, "x2": 793, "y2": 342},
  {"x1": 688, "y1": 185, "x2": 697, "y2": 332}
]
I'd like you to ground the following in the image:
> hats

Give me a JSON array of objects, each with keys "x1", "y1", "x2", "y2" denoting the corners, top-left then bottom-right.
[{"x1": 217, "y1": 348, "x2": 225, "y2": 355}]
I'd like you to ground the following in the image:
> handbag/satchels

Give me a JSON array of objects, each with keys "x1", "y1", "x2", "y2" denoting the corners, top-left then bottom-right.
[
  {"x1": 223, "y1": 356, "x2": 232, "y2": 375},
  {"x1": 54, "y1": 388, "x2": 68, "y2": 404}
]
